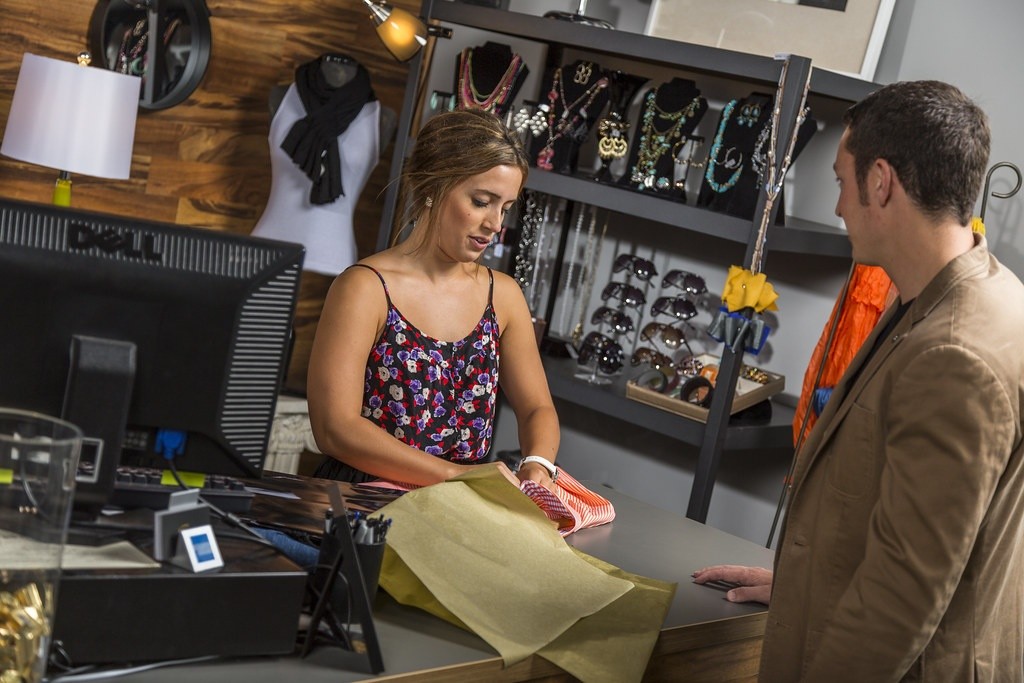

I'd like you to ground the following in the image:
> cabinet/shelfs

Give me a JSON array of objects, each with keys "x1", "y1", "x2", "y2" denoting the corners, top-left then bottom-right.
[{"x1": 374, "y1": 0, "x2": 887, "y2": 526}]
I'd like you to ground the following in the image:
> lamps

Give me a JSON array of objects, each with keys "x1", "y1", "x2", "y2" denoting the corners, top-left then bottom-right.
[
  {"x1": 1, "y1": 51, "x2": 141, "y2": 209},
  {"x1": 363, "y1": 0, "x2": 427, "y2": 63}
]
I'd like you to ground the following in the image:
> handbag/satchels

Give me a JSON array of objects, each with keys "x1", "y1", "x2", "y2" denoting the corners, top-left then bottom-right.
[{"x1": 512, "y1": 456, "x2": 616, "y2": 538}]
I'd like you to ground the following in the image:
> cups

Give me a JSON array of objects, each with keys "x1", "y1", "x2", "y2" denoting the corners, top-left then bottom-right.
[
  {"x1": 0, "y1": 408, "x2": 84, "y2": 683},
  {"x1": 306, "y1": 530, "x2": 385, "y2": 623}
]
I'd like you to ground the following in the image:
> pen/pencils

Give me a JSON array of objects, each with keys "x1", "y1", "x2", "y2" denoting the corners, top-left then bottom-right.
[{"x1": 323, "y1": 507, "x2": 393, "y2": 545}]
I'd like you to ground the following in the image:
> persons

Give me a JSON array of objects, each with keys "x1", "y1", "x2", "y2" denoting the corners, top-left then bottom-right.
[
  {"x1": 250, "y1": 52, "x2": 397, "y2": 277},
  {"x1": 304, "y1": 108, "x2": 560, "y2": 489},
  {"x1": 688, "y1": 81, "x2": 1024, "y2": 683}
]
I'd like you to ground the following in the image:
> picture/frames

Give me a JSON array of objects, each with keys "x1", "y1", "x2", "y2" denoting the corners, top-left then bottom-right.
[{"x1": 644, "y1": 0, "x2": 896, "y2": 83}]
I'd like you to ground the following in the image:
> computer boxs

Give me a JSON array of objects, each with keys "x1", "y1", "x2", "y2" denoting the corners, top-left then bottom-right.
[{"x1": 0, "y1": 504, "x2": 310, "y2": 656}]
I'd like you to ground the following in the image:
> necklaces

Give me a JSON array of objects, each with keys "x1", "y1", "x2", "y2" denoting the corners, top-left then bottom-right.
[
  {"x1": 457, "y1": 47, "x2": 525, "y2": 113},
  {"x1": 706, "y1": 97, "x2": 743, "y2": 193},
  {"x1": 706, "y1": 57, "x2": 813, "y2": 354},
  {"x1": 635, "y1": 88, "x2": 701, "y2": 182},
  {"x1": 536, "y1": 69, "x2": 611, "y2": 168}
]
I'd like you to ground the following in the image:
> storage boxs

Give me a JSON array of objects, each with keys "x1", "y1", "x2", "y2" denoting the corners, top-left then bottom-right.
[{"x1": 626, "y1": 352, "x2": 786, "y2": 424}]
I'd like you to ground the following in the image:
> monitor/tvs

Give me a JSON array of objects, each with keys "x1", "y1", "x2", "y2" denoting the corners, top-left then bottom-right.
[{"x1": 0, "y1": 196, "x2": 306, "y2": 531}]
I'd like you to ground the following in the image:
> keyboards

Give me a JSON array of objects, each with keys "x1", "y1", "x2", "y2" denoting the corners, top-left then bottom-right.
[{"x1": 0, "y1": 449, "x2": 256, "y2": 513}]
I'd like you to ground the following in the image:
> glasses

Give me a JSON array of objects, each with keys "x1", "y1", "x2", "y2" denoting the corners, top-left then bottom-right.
[
  {"x1": 650, "y1": 296, "x2": 698, "y2": 330},
  {"x1": 639, "y1": 322, "x2": 693, "y2": 356},
  {"x1": 661, "y1": 269, "x2": 708, "y2": 296},
  {"x1": 577, "y1": 344, "x2": 624, "y2": 376},
  {"x1": 630, "y1": 347, "x2": 675, "y2": 372},
  {"x1": 582, "y1": 331, "x2": 625, "y2": 359},
  {"x1": 612, "y1": 253, "x2": 658, "y2": 288},
  {"x1": 601, "y1": 282, "x2": 647, "y2": 317},
  {"x1": 590, "y1": 306, "x2": 635, "y2": 344}
]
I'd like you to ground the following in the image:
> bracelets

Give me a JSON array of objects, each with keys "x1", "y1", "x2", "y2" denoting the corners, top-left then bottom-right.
[{"x1": 518, "y1": 456, "x2": 558, "y2": 482}]
